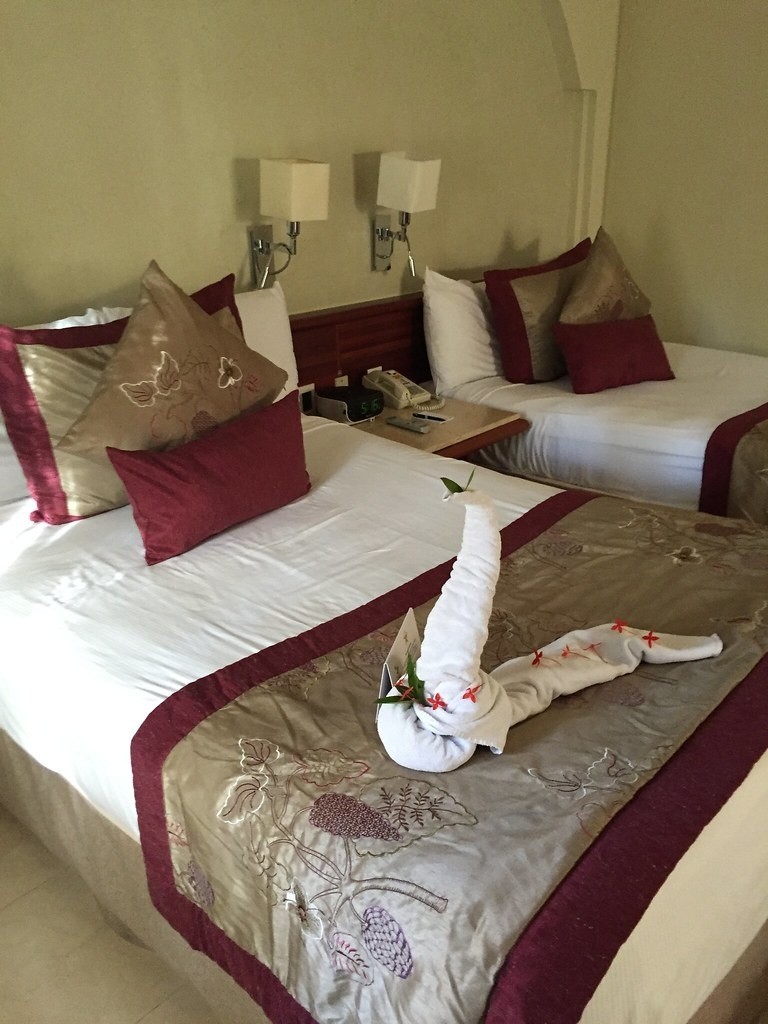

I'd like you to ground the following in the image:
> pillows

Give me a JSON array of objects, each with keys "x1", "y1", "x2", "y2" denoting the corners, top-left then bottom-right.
[
  {"x1": 423, "y1": 263, "x2": 504, "y2": 401},
  {"x1": 483, "y1": 236, "x2": 593, "y2": 384},
  {"x1": 550, "y1": 313, "x2": 676, "y2": 394},
  {"x1": 0, "y1": 273, "x2": 245, "y2": 524},
  {"x1": 557, "y1": 225, "x2": 651, "y2": 324},
  {"x1": 53, "y1": 259, "x2": 289, "y2": 468},
  {"x1": 0, "y1": 280, "x2": 299, "y2": 508},
  {"x1": 104, "y1": 389, "x2": 312, "y2": 567}
]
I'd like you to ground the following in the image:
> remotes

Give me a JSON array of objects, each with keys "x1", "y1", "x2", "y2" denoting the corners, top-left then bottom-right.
[{"x1": 386, "y1": 416, "x2": 431, "y2": 433}]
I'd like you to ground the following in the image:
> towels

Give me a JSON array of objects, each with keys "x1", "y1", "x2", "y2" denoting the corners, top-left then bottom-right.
[{"x1": 374, "y1": 483, "x2": 724, "y2": 774}]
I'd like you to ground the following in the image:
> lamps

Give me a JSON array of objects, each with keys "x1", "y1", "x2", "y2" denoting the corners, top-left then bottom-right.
[
  {"x1": 246, "y1": 156, "x2": 331, "y2": 291},
  {"x1": 372, "y1": 150, "x2": 443, "y2": 278}
]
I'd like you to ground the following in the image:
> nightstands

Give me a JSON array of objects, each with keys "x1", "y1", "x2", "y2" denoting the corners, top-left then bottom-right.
[{"x1": 349, "y1": 396, "x2": 530, "y2": 458}]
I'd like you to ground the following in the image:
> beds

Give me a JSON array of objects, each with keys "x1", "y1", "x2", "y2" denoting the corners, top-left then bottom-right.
[
  {"x1": 0, "y1": 412, "x2": 767, "y2": 1024},
  {"x1": 419, "y1": 342, "x2": 767, "y2": 524}
]
